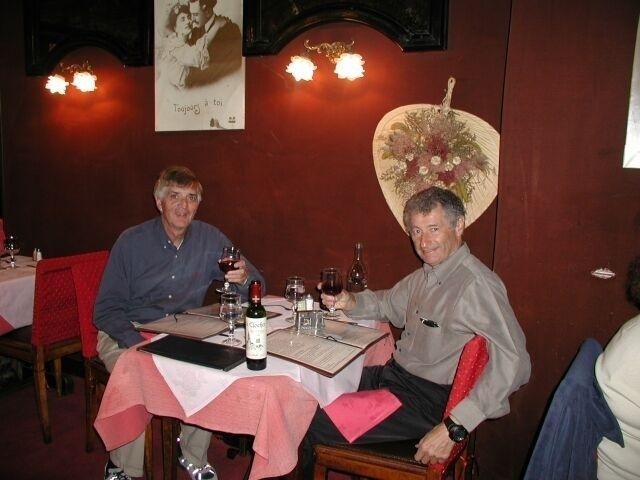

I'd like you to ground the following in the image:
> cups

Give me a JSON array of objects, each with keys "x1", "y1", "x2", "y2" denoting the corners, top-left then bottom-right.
[{"x1": 293, "y1": 311, "x2": 325, "y2": 333}]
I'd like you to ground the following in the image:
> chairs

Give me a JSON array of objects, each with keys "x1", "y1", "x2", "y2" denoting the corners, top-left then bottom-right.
[
  {"x1": 1, "y1": 249, "x2": 106, "y2": 444},
  {"x1": 321, "y1": 335, "x2": 490, "y2": 477},
  {"x1": 553, "y1": 335, "x2": 611, "y2": 480},
  {"x1": 67, "y1": 255, "x2": 186, "y2": 480}
]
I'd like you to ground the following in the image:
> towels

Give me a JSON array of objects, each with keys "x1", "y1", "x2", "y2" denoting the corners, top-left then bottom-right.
[{"x1": 321, "y1": 385, "x2": 404, "y2": 446}]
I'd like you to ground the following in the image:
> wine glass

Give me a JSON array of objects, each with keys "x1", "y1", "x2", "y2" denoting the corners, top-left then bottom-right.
[
  {"x1": 321, "y1": 267, "x2": 343, "y2": 320},
  {"x1": 219, "y1": 293, "x2": 243, "y2": 347},
  {"x1": 215, "y1": 246, "x2": 242, "y2": 295},
  {"x1": 284, "y1": 276, "x2": 305, "y2": 324},
  {"x1": 5, "y1": 238, "x2": 20, "y2": 269}
]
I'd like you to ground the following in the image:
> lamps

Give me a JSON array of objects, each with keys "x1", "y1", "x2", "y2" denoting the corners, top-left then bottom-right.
[
  {"x1": 282, "y1": 36, "x2": 368, "y2": 83},
  {"x1": 44, "y1": 52, "x2": 105, "y2": 97}
]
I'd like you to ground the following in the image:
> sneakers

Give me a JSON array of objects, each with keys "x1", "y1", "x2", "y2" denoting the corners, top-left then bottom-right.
[
  {"x1": 104, "y1": 459, "x2": 131, "y2": 480},
  {"x1": 177, "y1": 438, "x2": 218, "y2": 480}
]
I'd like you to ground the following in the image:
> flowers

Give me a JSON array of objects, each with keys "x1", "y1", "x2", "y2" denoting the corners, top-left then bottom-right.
[{"x1": 376, "y1": 106, "x2": 497, "y2": 220}]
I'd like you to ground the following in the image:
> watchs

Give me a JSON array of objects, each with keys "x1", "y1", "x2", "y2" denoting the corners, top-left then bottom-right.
[{"x1": 444, "y1": 415, "x2": 468, "y2": 444}]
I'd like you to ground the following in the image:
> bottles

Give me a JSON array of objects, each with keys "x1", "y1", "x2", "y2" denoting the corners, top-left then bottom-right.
[
  {"x1": 245, "y1": 280, "x2": 267, "y2": 371},
  {"x1": 346, "y1": 242, "x2": 368, "y2": 292},
  {"x1": 32, "y1": 248, "x2": 42, "y2": 261},
  {"x1": 305, "y1": 294, "x2": 313, "y2": 311}
]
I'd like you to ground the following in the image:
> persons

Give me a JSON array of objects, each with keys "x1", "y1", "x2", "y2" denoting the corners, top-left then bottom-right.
[
  {"x1": 183, "y1": 0, "x2": 242, "y2": 88},
  {"x1": 297, "y1": 184, "x2": 533, "y2": 480},
  {"x1": 92, "y1": 162, "x2": 267, "y2": 480},
  {"x1": 160, "y1": 3, "x2": 233, "y2": 89},
  {"x1": 595, "y1": 255, "x2": 639, "y2": 480}
]
